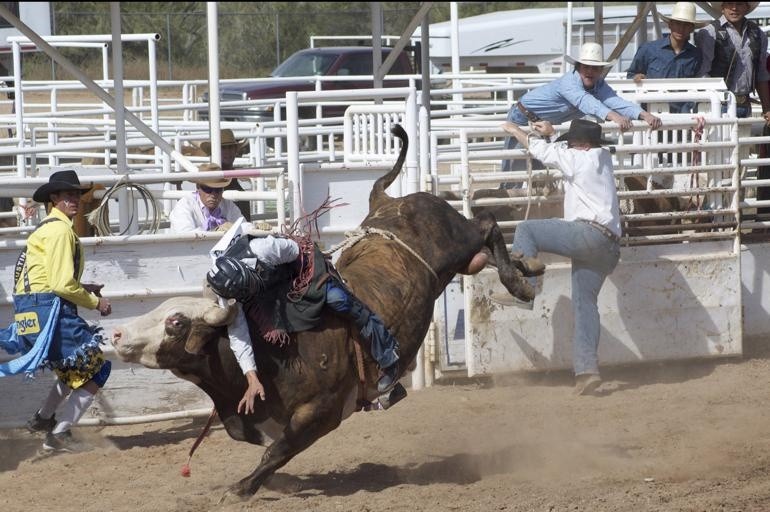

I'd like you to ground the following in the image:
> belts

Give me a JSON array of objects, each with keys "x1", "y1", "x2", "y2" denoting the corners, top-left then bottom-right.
[
  {"x1": 577, "y1": 216, "x2": 624, "y2": 247},
  {"x1": 516, "y1": 100, "x2": 548, "y2": 128}
]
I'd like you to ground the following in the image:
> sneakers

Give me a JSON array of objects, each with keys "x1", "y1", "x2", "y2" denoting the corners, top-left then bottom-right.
[
  {"x1": 27, "y1": 410, "x2": 61, "y2": 431},
  {"x1": 42, "y1": 429, "x2": 95, "y2": 454},
  {"x1": 490, "y1": 288, "x2": 537, "y2": 310},
  {"x1": 377, "y1": 344, "x2": 407, "y2": 392},
  {"x1": 571, "y1": 370, "x2": 602, "y2": 397}
]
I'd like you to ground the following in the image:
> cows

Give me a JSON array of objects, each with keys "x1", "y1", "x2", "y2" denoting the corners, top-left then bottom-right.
[
  {"x1": 621, "y1": 175, "x2": 715, "y2": 247},
  {"x1": 110, "y1": 124, "x2": 547, "y2": 506}
]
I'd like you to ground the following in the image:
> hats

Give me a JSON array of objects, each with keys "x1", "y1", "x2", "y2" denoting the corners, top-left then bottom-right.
[
  {"x1": 183, "y1": 163, "x2": 236, "y2": 191},
  {"x1": 657, "y1": 0, "x2": 712, "y2": 30},
  {"x1": 710, "y1": 0, "x2": 762, "y2": 17},
  {"x1": 32, "y1": 169, "x2": 96, "y2": 203},
  {"x1": 564, "y1": 40, "x2": 612, "y2": 68},
  {"x1": 200, "y1": 129, "x2": 248, "y2": 154},
  {"x1": 552, "y1": 119, "x2": 616, "y2": 147}
]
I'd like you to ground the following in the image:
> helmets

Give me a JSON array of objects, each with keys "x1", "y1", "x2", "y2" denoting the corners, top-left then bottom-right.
[{"x1": 208, "y1": 257, "x2": 268, "y2": 303}]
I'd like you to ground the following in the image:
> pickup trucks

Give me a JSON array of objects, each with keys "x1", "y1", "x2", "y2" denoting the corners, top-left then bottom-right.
[{"x1": 194, "y1": 46, "x2": 494, "y2": 154}]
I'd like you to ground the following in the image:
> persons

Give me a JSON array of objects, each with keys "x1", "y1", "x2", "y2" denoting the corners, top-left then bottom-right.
[
  {"x1": 1, "y1": 196, "x2": 19, "y2": 230},
  {"x1": 168, "y1": 163, "x2": 272, "y2": 231},
  {"x1": 501, "y1": 117, "x2": 621, "y2": 397},
  {"x1": 0, "y1": 169, "x2": 113, "y2": 448},
  {"x1": 500, "y1": 42, "x2": 662, "y2": 187},
  {"x1": 694, "y1": 0, "x2": 768, "y2": 242},
  {"x1": 627, "y1": 1, "x2": 716, "y2": 166},
  {"x1": 752, "y1": 53, "x2": 770, "y2": 235},
  {"x1": 201, "y1": 129, "x2": 250, "y2": 223},
  {"x1": 207, "y1": 231, "x2": 409, "y2": 414}
]
